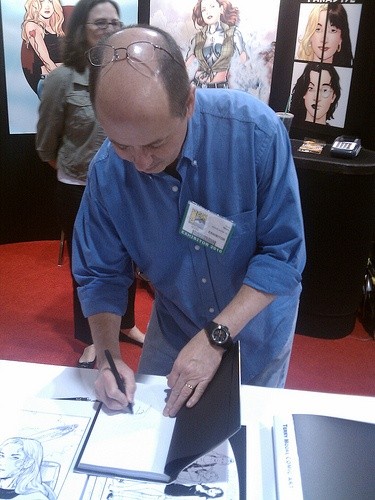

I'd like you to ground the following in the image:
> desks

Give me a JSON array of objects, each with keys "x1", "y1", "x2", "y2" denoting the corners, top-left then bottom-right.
[
  {"x1": 284, "y1": 138, "x2": 375, "y2": 339},
  {"x1": 0, "y1": 360, "x2": 375, "y2": 500}
]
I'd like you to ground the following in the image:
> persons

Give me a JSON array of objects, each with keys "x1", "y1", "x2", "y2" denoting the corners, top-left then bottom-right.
[
  {"x1": 71, "y1": 25, "x2": 307, "y2": 417},
  {"x1": 36, "y1": 0, "x2": 146, "y2": 368}
]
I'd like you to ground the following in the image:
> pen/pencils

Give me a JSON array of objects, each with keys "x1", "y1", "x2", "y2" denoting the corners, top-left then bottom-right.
[
  {"x1": 104, "y1": 349, "x2": 134, "y2": 415},
  {"x1": 53, "y1": 396, "x2": 93, "y2": 401}
]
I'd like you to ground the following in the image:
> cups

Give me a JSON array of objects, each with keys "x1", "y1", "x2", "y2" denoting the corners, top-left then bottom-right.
[{"x1": 276, "y1": 112, "x2": 294, "y2": 135}]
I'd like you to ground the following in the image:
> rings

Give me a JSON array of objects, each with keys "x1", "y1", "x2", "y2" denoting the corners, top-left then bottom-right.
[{"x1": 185, "y1": 383, "x2": 194, "y2": 390}]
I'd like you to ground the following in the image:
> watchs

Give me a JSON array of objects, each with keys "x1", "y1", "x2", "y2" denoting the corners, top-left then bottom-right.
[{"x1": 203, "y1": 320, "x2": 235, "y2": 351}]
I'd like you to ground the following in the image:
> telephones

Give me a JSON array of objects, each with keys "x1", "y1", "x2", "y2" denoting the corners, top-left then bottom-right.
[{"x1": 331, "y1": 137, "x2": 362, "y2": 157}]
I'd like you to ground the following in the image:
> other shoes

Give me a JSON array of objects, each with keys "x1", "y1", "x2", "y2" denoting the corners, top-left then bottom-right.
[
  {"x1": 119, "y1": 332, "x2": 143, "y2": 346},
  {"x1": 77, "y1": 355, "x2": 96, "y2": 368}
]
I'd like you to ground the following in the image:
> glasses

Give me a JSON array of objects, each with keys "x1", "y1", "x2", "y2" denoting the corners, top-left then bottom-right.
[
  {"x1": 84, "y1": 41, "x2": 186, "y2": 66},
  {"x1": 87, "y1": 19, "x2": 121, "y2": 28}
]
